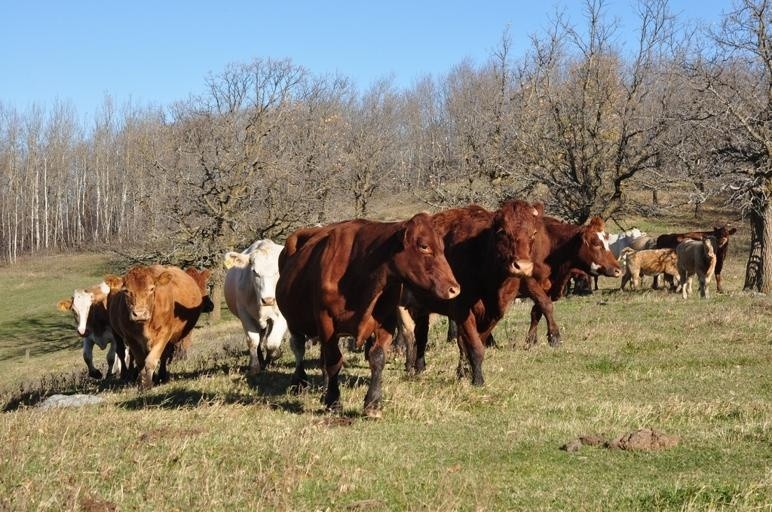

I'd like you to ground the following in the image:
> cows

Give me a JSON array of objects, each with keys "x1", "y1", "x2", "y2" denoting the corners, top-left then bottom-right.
[
  {"x1": 620, "y1": 225, "x2": 736, "y2": 300},
  {"x1": 55, "y1": 262, "x2": 214, "y2": 390},
  {"x1": 222, "y1": 237, "x2": 287, "y2": 374},
  {"x1": 397, "y1": 198, "x2": 545, "y2": 388},
  {"x1": 277, "y1": 211, "x2": 463, "y2": 421},
  {"x1": 445, "y1": 212, "x2": 622, "y2": 349},
  {"x1": 592, "y1": 230, "x2": 622, "y2": 291}
]
